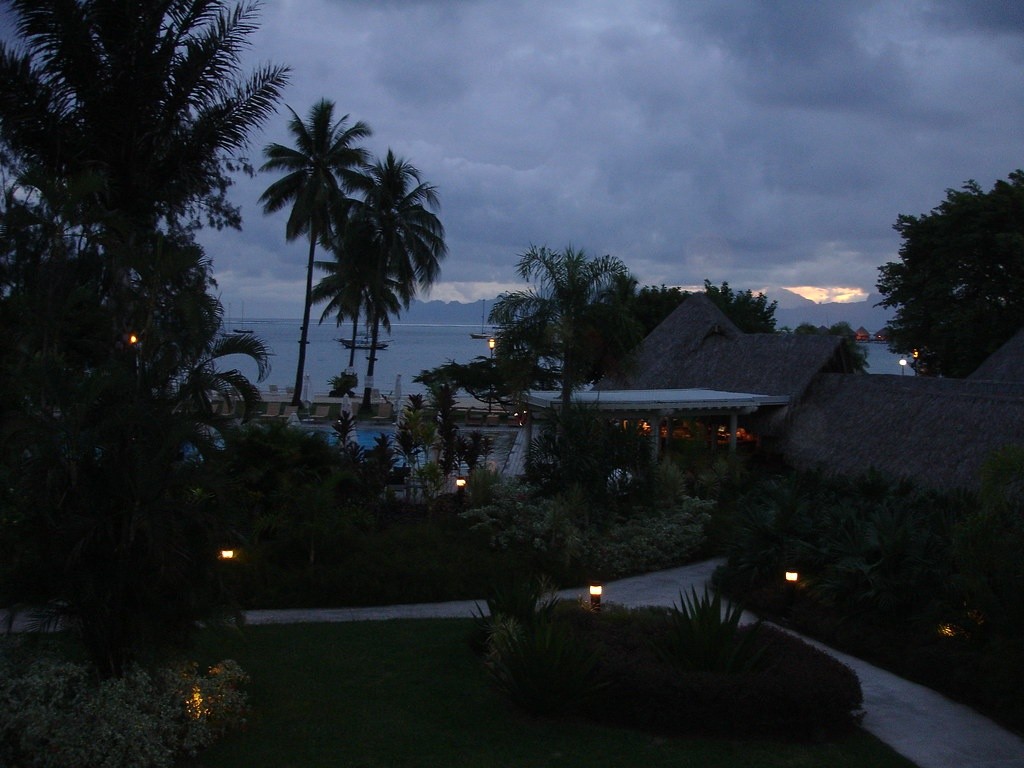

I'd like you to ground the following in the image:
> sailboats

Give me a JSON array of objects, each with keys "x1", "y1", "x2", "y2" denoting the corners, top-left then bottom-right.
[
  {"x1": 233, "y1": 301, "x2": 254, "y2": 334},
  {"x1": 222, "y1": 304, "x2": 245, "y2": 338},
  {"x1": 340, "y1": 313, "x2": 389, "y2": 350},
  {"x1": 469, "y1": 299, "x2": 491, "y2": 339}
]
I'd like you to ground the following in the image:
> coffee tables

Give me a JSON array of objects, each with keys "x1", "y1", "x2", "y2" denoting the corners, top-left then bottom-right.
[{"x1": 486, "y1": 415, "x2": 499, "y2": 426}]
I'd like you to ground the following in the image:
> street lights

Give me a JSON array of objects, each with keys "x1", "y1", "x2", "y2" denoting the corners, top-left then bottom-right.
[
  {"x1": 900, "y1": 359, "x2": 907, "y2": 376},
  {"x1": 488, "y1": 339, "x2": 495, "y2": 414}
]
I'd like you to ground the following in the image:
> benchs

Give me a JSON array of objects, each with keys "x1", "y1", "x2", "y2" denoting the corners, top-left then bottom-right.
[
  {"x1": 465, "y1": 410, "x2": 482, "y2": 427},
  {"x1": 507, "y1": 410, "x2": 520, "y2": 428}
]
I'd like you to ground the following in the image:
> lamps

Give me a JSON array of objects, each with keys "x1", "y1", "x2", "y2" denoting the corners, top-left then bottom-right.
[
  {"x1": 589, "y1": 582, "x2": 603, "y2": 595},
  {"x1": 456, "y1": 476, "x2": 467, "y2": 485},
  {"x1": 785, "y1": 568, "x2": 798, "y2": 581},
  {"x1": 220, "y1": 544, "x2": 235, "y2": 559}
]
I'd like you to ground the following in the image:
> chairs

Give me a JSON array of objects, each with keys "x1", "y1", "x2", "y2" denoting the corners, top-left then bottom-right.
[
  {"x1": 351, "y1": 402, "x2": 360, "y2": 421},
  {"x1": 371, "y1": 404, "x2": 391, "y2": 425},
  {"x1": 279, "y1": 405, "x2": 299, "y2": 422},
  {"x1": 261, "y1": 402, "x2": 282, "y2": 421},
  {"x1": 286, "y1": 387, "x2": 295, "y2": 399},
  {"x1": 268, "y1": 385, "x2": 280, "y2": 396},
  {"x1": 310, "y1": 406, "x2": 329, "y2": 424}
]
